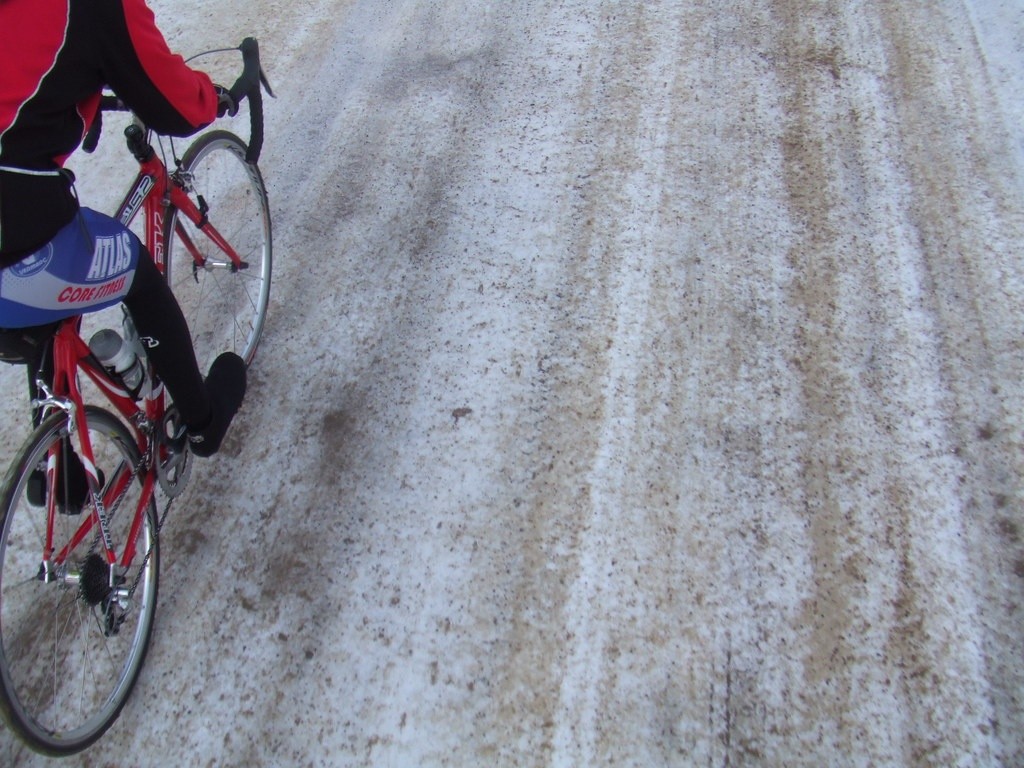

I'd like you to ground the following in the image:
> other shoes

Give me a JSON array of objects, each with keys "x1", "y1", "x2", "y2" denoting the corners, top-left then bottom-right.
[
  {"x1": 185, "y1": 352, "x2": 247, "y2": 457},
  {"x1": 27, "y1": 452, "x2": 105, "y2": 507}
]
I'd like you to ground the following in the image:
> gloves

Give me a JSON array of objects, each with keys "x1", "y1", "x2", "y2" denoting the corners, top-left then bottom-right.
[{"x1": 212, "y1": 83, "x2": 238, "y2": 118}]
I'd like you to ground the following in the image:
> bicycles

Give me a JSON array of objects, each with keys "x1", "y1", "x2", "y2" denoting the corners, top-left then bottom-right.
[{"x1": 0, "y1": 37, "x2": 279, "y2": 757}]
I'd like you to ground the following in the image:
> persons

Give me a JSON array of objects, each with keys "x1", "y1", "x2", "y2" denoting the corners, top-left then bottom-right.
[{"x1": 0, "y1": 0, "x2": 249, "y2": 512}]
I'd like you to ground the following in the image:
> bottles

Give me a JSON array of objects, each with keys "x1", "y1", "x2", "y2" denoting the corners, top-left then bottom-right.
[
  {"x1": 89, "y1": 328, "x2": 151, "y2": 399},
  {"x1": 121, "y1": 304, "x2": 148, "y2": 370}
]
